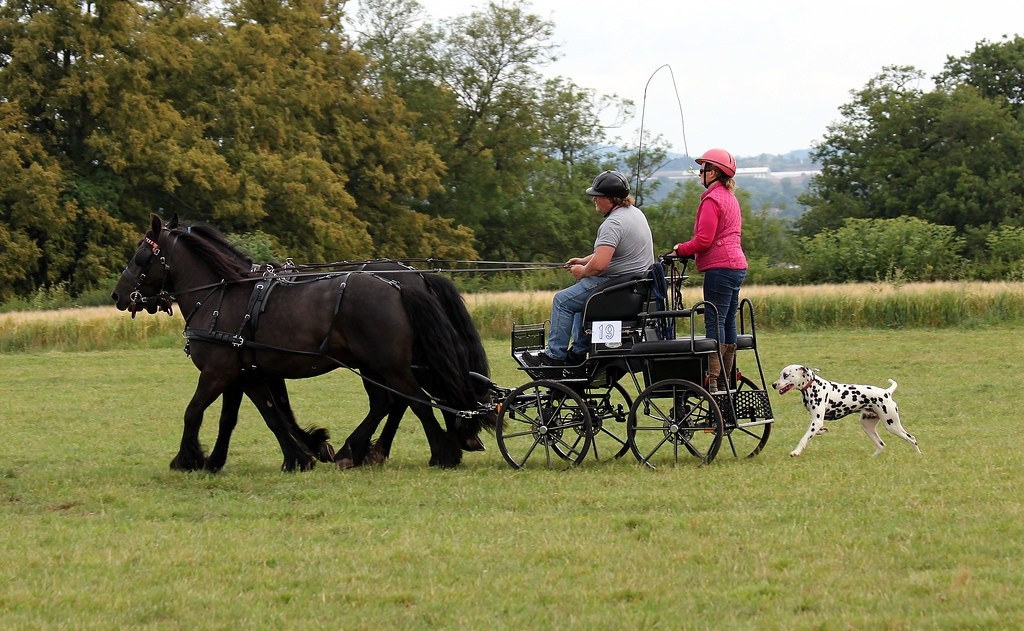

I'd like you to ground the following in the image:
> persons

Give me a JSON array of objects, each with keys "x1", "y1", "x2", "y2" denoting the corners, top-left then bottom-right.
[
  {"x1": 664, "y1": 149, "x2": 750, "y2": 393},
  {"x1": 521, "y1": 170, "x2": 655, "y2": 379}
]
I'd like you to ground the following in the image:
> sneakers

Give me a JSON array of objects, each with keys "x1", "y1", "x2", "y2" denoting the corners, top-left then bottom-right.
[
  {"x1": 522, "y1": 351, "x2": 564, "y2": 378},
  {"x1": 563, "y1": 346, "x2": 586, "y2": 376}
]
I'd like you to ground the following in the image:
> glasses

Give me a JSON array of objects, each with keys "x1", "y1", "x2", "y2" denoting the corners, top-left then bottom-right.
[{"x1": 700, "y1": 169, "x2": 711, "y2": 174}]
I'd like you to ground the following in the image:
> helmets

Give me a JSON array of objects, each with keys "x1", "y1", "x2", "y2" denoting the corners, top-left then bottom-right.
[
  {"x1": 586, "y1": 170, "x2": 630, "y2": 198},
  {"x1": 695, "y1": 148, "x2": 737, "y2": 176}
]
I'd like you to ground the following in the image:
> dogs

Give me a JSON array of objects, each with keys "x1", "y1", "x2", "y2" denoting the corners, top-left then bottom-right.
[{"x1": 772, "y1": 364, "x2": 922, "y2": 459}]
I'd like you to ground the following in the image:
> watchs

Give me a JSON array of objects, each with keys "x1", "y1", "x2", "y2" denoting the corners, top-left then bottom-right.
[{"x1": 673, "y1": 244, "x2": 679, "y2": 257}]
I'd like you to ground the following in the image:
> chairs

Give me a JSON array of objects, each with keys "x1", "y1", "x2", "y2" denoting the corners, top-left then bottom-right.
[{"x1": 580, "y1": 272, "x2": 652, "y2": 361}]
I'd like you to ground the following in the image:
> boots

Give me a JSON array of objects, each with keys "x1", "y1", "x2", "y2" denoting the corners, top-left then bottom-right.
[
  {"x1": 705, "y1": 342, "x2": 728, "y2": 391},
  {"x1": 717, "y1": 343, "x2": 737, "y2": 390}
]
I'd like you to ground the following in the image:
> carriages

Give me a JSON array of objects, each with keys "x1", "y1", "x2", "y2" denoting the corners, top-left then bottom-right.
[{"x1": 111, "y1": 211, "x2": 777, "y2": 472}]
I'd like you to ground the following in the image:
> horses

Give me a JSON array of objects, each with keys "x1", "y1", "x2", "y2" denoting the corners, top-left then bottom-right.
[{"x1": 108, "y1": 211, "x2": 510, "y2": 475}]
